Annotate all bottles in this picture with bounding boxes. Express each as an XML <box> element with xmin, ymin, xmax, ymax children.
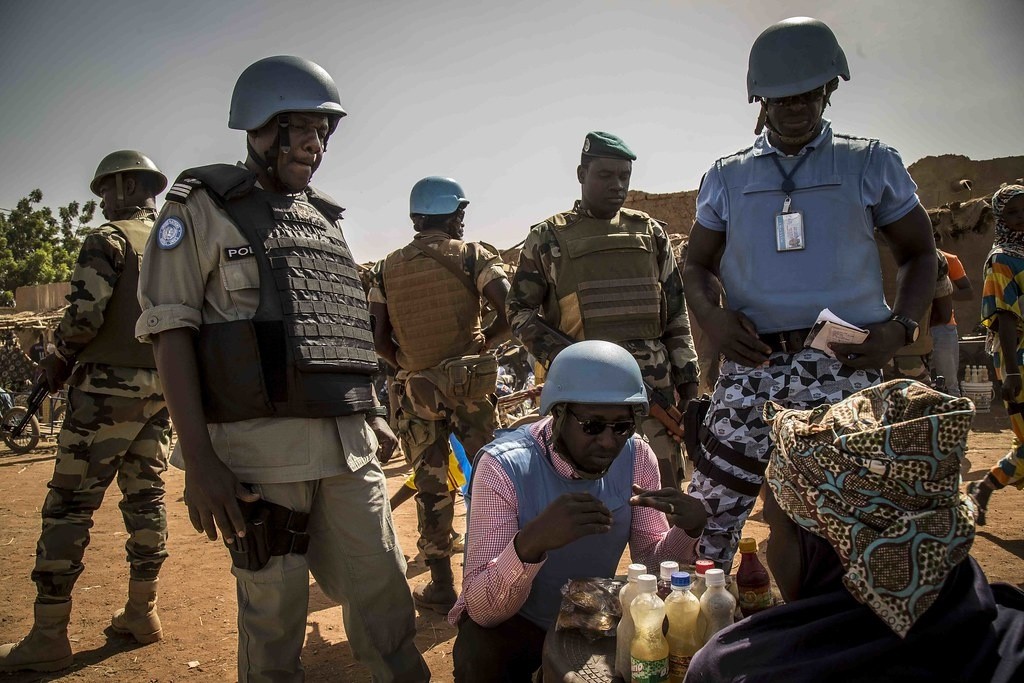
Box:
<box><xmin>700</xmin><ymin>569</ymin><xmax>736</xmax><ymax>643</ymax></box>
<box><xmin>655</xmin><ymin>561</ymin><xmax>679</xmax><ymax>637</ymax></box>
<box><xmin>664</xmin><ymin>571</ymin><xmax>704</xmax><ymax>683</ymax></box>
<box><xmin>614</xmin><ymin>564</ymin><xmax>646</xmax><ymax>683</ymax></box>
<box><xmin>965</xmin><ymin>365</ymin><xmax>988</xmax><ymax>383</ymax></box>
<box><xmin>736</xmin><ymin>538</ymin><xmax>773</xmax><ymax>618</ymax></box>
<box><xmin>630</xmin><ymin>574</ymin><xmax>670</xmax><ymax>683</ymax></box>
<box><xmin>689</xmin><ymin>560</ymin><xmax>714</xmax><ymax>637</ymax></box>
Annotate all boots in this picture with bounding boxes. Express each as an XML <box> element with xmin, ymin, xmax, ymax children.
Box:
<box><xmin>110</xmin><ymin>578</ymin><xmax>164</xmax><ymax>644</ymax></box>
<box><xmin>413</xmin><ymin>556</ymin><xmax>458</xmax><ymax>615</ymax></box>
<box><xmin>0</xmin><ymin>599</ymin><xmax>74</xmax><ymax>672</ymax></box>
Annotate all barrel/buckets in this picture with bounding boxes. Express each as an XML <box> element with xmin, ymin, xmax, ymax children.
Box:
<box><xmin>960</xmin><ymin>381</ymin><xmax>995</xmax><ymax>413</ymax></box>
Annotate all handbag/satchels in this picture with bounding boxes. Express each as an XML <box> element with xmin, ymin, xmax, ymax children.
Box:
<box><xmin>419</xmin><ymin>355</ymin><xmax>499</xmax><ymax>397</ymax></box>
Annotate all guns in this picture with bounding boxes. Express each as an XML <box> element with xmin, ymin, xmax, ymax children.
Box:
<box><xmin>517</xmin><ymin>305</ymin><xmax>685</xmax><ymax>438</ymax></box>
<box><xmin>4</xmin><ymin>371</ymin><xmax>50</xmax><ymax>439</ymax></box>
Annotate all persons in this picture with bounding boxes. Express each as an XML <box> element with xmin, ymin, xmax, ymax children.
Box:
<box><xmin>966</xmin><ymin>183</ymin><xmax>1024</xmax><ymax>526</ymax></box>
<box><xmin>505</xmin><ymin>131</ymin><xmax>701</xmax><ymax>526</ymax></box>
<box><xmin>788</xmin><ymin>235</ymin><xmax>802</xmax><ymax>246</ymax></box>
<box><xmin>29</xmin><ymin>333</ymin><xmax>50</xmax><ymax>362</ymax></box>
<box><xmin>679</xmin><ymin>20</ymin><xmax>937</xmax><ymax>617</ymax></box>
<box><xmin>136</xmin><ymin>53</ymin><xmax>436</xmax><ymax>683</ymax></box>
<box><xmin>445</xmin><ymin>337</ymin><xmax>708</xmax><ymax>683</ymax></box>
<box><xmin>0</xmin><ymin>149</ymin><xmax>175</xmax><ymax>675</ymax></box>
<box><xmin>924</xmin><ymin>225</ymin><xmax>974</xmax><ymax>395</ymax></box>
<box><xmin>366</xmin><ymin>178</ymin><xmax>513</xmax><ymax>618</ymax></box>
<box><xmin>681</xmin><ymin>372</ymin><xmax>1024</xmax><ymax>683</ymax></box>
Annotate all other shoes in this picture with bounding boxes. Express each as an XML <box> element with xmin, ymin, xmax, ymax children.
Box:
<box><xmin>965</xmin><ymin>481</ymin><xmax>991</xmax><ymax>525</ymax></box>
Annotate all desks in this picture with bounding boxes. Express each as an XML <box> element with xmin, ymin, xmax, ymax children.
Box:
<box><xmin>540</xmin><ymin>576</ymin><xmax>785</xmax><ymax>683</ymax></box>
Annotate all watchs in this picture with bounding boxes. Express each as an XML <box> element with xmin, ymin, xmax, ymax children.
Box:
<box><xmin>890</xmin><ymin>314</ymin><xmax>921</xmax><ymax>347</ymax></box>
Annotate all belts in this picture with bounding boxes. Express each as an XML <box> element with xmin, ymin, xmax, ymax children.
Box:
<box><xmin>755</xmin><ymin>328</ymin><xmax>810</xmax><ymax>354</ymax></box>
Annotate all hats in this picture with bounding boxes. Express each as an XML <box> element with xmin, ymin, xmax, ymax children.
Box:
<box><xmin>581</xmin><ymin>132</ymin><xmax>637</xmax><ymax>161</ymax></box>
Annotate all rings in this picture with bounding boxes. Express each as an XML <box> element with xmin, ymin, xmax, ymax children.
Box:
<box><xmin>668</xmin><ymin>504</ymin><xmax>675</xmax><ymax>513</ymax></box>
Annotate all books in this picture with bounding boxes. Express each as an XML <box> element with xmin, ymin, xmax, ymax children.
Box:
<box><xmin>803</xmin><ymin>307</ymin><xmax>870</xmax><ymax>360</ymax></box>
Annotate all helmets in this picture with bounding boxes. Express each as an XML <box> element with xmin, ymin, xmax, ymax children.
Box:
<box><xmin>539</xmin><ymin>339</ymin><xmax>651</xmax><ymax>417</ymax></box>
<box><xmin>746</xmin><ymin>17</ymin><xmax>850</xmax><ymax>102</ymax></box>
<box><xmin>90</xmin><ymin>149</ymin><xmax>168</xmax><ymax>196</ymax></box>
<box><xmin>228</xmin><ymin>56</ymin><xmax>347</xmax><ymax>130</ymax></box>
<box><xmin>409</xmin><ymin>177</ymin><xmax>469</xmax><ymax>214</ymax></box>
<box><xmin>748</xmin><ymin>17</ymin><xmax>850</xmax><ymax>103</ymax></box>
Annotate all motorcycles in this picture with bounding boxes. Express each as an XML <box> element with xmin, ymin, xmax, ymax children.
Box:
<box><xmin>0</xmin><ymin>386</ymin><xmax>40</xmax><ymax>453</ymax></box>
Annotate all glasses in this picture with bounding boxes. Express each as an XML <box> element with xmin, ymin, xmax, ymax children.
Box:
<box><xmin>565</xmin><ymin>408</ymin><xmax>635</xmax><ymax>437</ymax></box>
<box><xmin>766</xmin><ymin>83</ymin><xmax>827</xmax><ymax>107</ymax></box>
